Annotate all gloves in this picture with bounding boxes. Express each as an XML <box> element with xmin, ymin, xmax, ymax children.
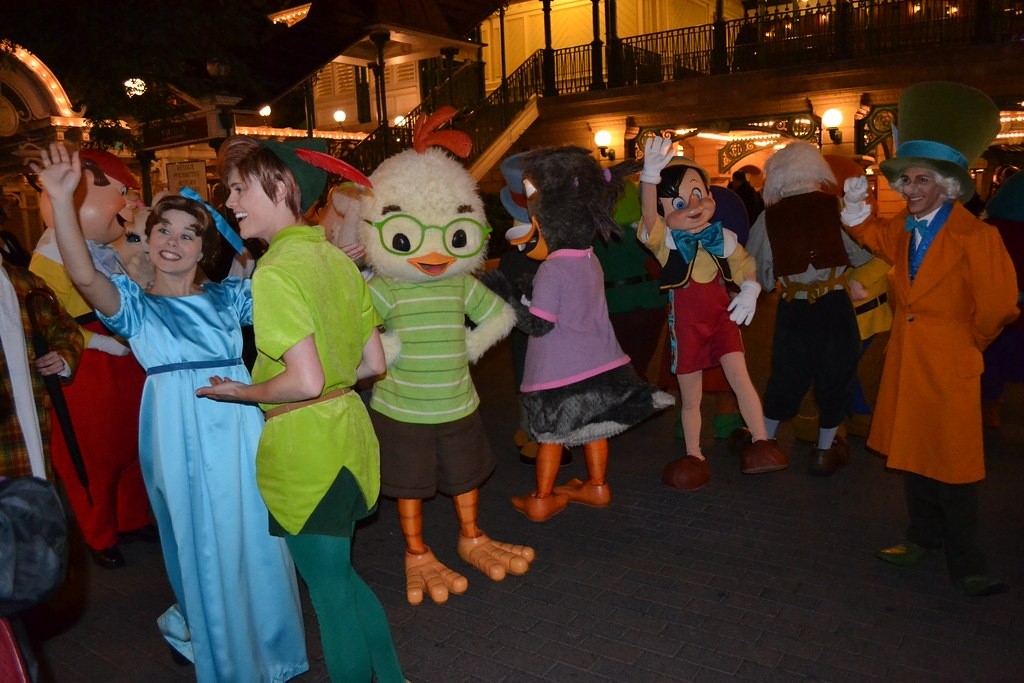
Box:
<box><xmin>843</xmin><ymin>176</ymin><xmax>869</xmax><ymax>203</ymax></box>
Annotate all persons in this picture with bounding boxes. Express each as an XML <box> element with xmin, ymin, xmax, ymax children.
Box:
<box><xmin>962</xmin><ymin>165</ymin><xmax>1017</xmax><ymax>221</ymax></box>
<box><xmin>725</xmin><ymin>168</ymin><xmax>756</xmax><ymax>221</ymax></box>
<box><xmin>0</xmin><ymin>207</ymin><xmax>84</xmax><ymax>493</ymax></box>
<box><xmin>36</xmin><ymin>140</ymin><xmax>365</xmax><ymax>683</ymax></box>
<box><xmin>735</xmin><ymin>22</ymin><xmax>762</xmax><ymax>63</ymax></box>
<box><xmin>195</xmin><ymin>138</ymin><xmax>414</xmax><ymax>683</ymax></box>
<box><xmin>0</xmin><ymin>476</ymin><xmax>67</xmax><ymax>683</ymax></box>
<box><xmin>840</xmin><ymin>80</ymin><xmax>1020</xmax><ymax>599</ymax></box>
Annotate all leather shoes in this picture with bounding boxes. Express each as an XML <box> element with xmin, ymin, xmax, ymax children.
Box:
<box><xmin>93</xmin><ymin>544</ymin><xmax>124</xmax><ymax>569</ymax></box>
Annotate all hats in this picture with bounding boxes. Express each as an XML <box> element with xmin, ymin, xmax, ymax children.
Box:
<box><xmin>879</xmin><ymin>81</ymin><xmax>1001</xmax><ymax>204</ymax></box>
<box><xmin>258</xmin><ymin>137</ymin><xmax>373</xmax><ymax>212</ymax></box>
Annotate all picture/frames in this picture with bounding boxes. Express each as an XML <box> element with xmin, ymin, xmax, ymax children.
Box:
<box><xmin>166</xmin><ymin>160</ymin><xmax>209</xmax><ymax>203</ymax></box>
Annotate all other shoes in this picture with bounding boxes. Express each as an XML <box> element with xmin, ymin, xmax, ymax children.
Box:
<box><xmin>877</xmin><ymin>542</ymin><xmax>930</xmax><ymax>568</ymax></box>
<box><xmin>956</xmin><ymin>574</ymin><xmax>1009</xmax><ymax>595</ymax></box>
<box><xmin>809</xmin><ymin>444</ymin><xmax>849</xmax><ymax>475</ymax></box>
<box><xmin>729</xmin><ymin>427</ymin><xmax>753</xmax><ymax>450</ymax></box>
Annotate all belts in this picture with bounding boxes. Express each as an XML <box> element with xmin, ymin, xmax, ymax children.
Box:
<box><xmin>265</xmin><ymin>386</ymin><xmax>353</xmax><ymax>420</ymax></box>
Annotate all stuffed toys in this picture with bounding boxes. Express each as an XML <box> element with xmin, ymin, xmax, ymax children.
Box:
<box><xmin>27</xmin><ymin>145</ymin><xmax>177</xmax><ymax>571</ymax></box>
<box><xmin>356</xmin><ymin>107</ymin><xmax>537</xmax><ymax>606</ymax></box>
<box><xmin>105</xmin><ymin>192</ymin><xmax>174</xmax><ymax>292</ymax></box>
<box><xmin>303</xmin><ymin>179</ymin><xmax>372</xmax><ymax>273</ymax></box>
<box><xmin>637</xmin><ymin>133</ymin><xmax>787</xmax><ymax>492</ymax></box>
<box><xmin>473</xmin><ymin>143</ymin><xmax>676</xmax><ymax>522</ymax></box>
<box><xmin>744</xmin><ymin>141</ymin><xmax>862</xmax><ymax>476</ymax></box>
<box><xmin>822</xmin><ymin>154</ymin><xmax>892</xmax><ymax>460</ymax></box>
<box><xmin>982</xmin><ymin>171</ymin><xmax>1024</xmax><ymax>428</ymax></box>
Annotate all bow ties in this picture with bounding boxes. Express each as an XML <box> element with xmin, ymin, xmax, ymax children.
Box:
<box><xmin>904</xmin><ymin>214</ymin><xmax>928</xmax><ymax>237</ymax></box>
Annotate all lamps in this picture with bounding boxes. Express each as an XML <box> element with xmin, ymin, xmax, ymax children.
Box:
<box><xmin>334</xmin><ymin>110</ymin><xmax>346</xmax><ymax>133</ymax></box>
<box><xmin>595</xmin><ymin>129</ymin><xmax>617</xmax><ymax>162</ymax></box>
<box><xmin>394</xmin><ymin>116</ymin><xmax>408</xmax><ymax>141</ymax></box>
<box><xmin>259</xmin><ymin>106</ymin><xmax>271</xmax><ymax>125</ymax></box>
<box><xmin>823</xmin><ymin>108</ymin><xmax>843</xmax><ymax>144</ymax></box>
